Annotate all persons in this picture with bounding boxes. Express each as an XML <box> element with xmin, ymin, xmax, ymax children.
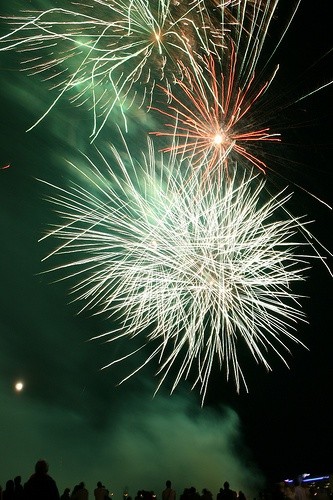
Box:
<box><xmin>180</xmin><ymin>481</ymin><xmax>246</xmax><ymax>500</ymax></box>
<box><xmin>60</xmin><ymin>487</ymin><xmax>71</xmax><ymax>500</ymax></box>
<box><xmin>94</xmin><ymin>481</ymin><xmax>107</xmax><ymax>500</ymax></box>
<box><xmin>122</xmin><ymin>488</ymin><xmax>158</xmax><ymax>500</ymax></box>
<box><xmin>0</xmin><ymin>479</ymin><xmax>19</xmax><ymax>500</ymax></box>
<box><xmin>13</xmin><ymin>475</ymin><xmax>24</xmax><ymax>500</ymax></box>
<box><xmin>79</xmin><ymin>481</ymin><xmax>89</xmax><ymax>500</ymax></box>
<box><xmin>162</xmin><ymin>480</ymin><xmax>177</xmax><ymax>500</ymax></box>
<box><xmin>102</xmin><ymin>485</ymin><xmax>112</xmax><ymax>500</ymax></box>
<box><xmin>70</xmin><ymin>484</ymin><xmax>86</xmax><ymax>500</ymax></box>
<box><xmin>254</xmin><ymin>474</ymin><xmax>333</xmax><ymax>500</ymax></box>
<box><xmin>24</xmin><ymin>458</ymin><xmax>60</xmax><ymax>500</ymax></box>
